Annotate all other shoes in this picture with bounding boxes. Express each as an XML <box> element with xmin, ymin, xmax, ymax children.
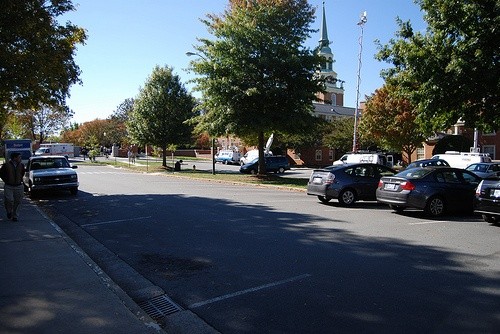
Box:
<box><xmin>7</xmin><ymin>216</ymin><xmax>18</xmax><ymax>222</ymax></box>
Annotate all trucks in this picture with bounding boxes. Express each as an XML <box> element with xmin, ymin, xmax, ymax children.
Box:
<box><xmin>73</xmin><ymin>146</ymin><xmax>82</xmax><ymax>157</ymax></box>
<box><xmin>333</xmin><ymin>153</ymin><xmax>394</xmax><ymax>169</ymax></box>
<box><xmin>239</xmin><ymin>149</ymin><xmax>273</xmax><ymax>166</ymax></box>
<box><xmin>35</xmin><ymin>142</ymin><xmax>75</xmax><ymax>161</ymax></box>
<box><xmin>4</xmin><ymin>138</ymin><xmax>33</xmax><ymax>166</ymax></box>
<box><xmin>214</xmin><ymin>150</ymin><xmax>241</xmax><ymax>165</ymax></box>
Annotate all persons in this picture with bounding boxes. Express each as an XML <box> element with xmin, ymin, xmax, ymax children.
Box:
<box><xmin>0</xmin><ymin>152</ymin><xmax>25</xmax><ymax>222</ymax></box>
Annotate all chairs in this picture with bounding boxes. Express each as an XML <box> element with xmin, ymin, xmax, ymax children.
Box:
<box><xmin>31</xmin><ymin>163</ymin><xmax>42</xmax><ymax>170</ymax></box>
<box><xmin>355</xmin><ymin>168</ymin><xmax>371</xmax><ymax>176</ymax></box>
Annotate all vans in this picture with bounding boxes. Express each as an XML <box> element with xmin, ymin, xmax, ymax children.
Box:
<box><xmin>239</xmin><ymin>154</ymin><xmax>290</xmax><ymax>174</ymax></box>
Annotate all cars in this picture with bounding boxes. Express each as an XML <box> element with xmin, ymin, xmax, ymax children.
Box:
<box><xmin>306</xmin><ymin>162</ymin><xmax>399</xmax><ymax>208</ymax></box>
<box><xmin>23</xmin><ymin>155</ymin><xmax>78</xmax><ymax>200</ymax></box>
<box><xmin>376</xmin><ymin>150</ymin><xmax>500</xmax><ymax>224</ymax></box>
